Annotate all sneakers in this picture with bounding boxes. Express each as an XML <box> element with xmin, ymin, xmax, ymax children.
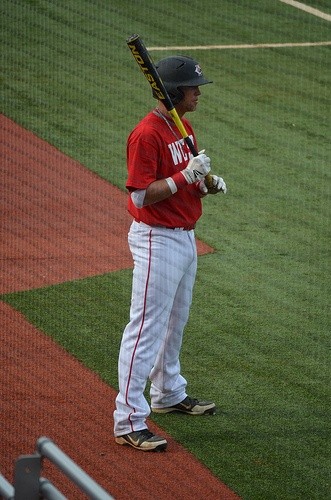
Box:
<box><xmin>115</xmin><ymin>429</ymin><xmax>168</xmax><ymax>452</ymax></box>
<box><xmin>152</xmin><ymin>396</ymin><xmax>216</xmax><ymax>416</ymax></box>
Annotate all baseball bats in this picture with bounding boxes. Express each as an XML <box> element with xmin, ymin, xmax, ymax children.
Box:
<box><xmin>127</xmin><ymin>33</ymin><xmax>220</xmax><ymax>193</ymax></box>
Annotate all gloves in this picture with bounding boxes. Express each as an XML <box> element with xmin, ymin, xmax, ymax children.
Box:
<box><xmin>199</xmin><ymin>175</ymin><xmax>227</xmax><ymax>194</ymax></box>
<box><xmin>180</xmin><ymin>149</ymin><xmax>211</xmax><ymax>185</ymax></box>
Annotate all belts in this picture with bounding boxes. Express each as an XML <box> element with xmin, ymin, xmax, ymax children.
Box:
<box><xmin>166</xmin><ymin>227</ymin><xmax>193</xmax><ymax>231</ymax></box>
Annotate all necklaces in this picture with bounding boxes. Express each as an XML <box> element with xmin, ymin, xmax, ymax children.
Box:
<box><xmin>155</xmin><ymin>107</ymin><xmax>189</xmax><ymax>154</ymax></box>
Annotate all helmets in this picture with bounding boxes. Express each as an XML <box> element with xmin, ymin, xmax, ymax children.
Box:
<box><xmin>154</xmin><ymin>55</ymin><xmax>213</xmax><ymax>102</ymax></box>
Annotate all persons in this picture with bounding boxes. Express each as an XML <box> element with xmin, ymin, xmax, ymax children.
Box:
<box><xmin>113</xmin><ymin>57</ymin><xmax>228</xmax><ymax>451</ymax></box>
